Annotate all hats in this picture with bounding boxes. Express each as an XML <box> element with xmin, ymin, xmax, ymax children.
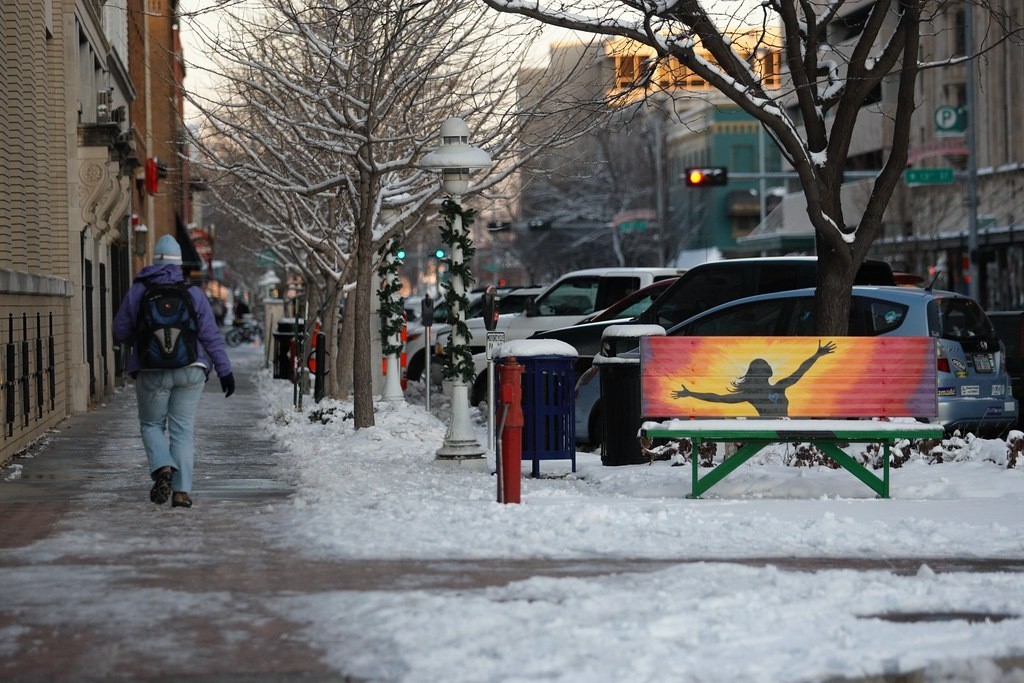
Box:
<box><xmin>153</xmin><ymin>233</ymin><xmax>182</xmax><ymax>265</ymax></box>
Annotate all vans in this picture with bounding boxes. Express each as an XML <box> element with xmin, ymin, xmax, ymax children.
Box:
<box><xmin>505</xmin><ymin>266</ymin><xmax>690</xmax><ymax>342</ymax></box>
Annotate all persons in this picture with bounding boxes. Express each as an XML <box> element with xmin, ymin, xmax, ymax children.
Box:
<box><xmin>233</xmin><ymin>295</ymin><xmax>250</xmax><ymax>319</ymax></box>
<box><xmin>208</xmin><ymin>296</ymin><xmax>228</xmax><ymax>327</ymax></box>
<box><xmin>112</xmin><ymin>234</ymin><xmax>235</xmax><ymax>508</ymax></box>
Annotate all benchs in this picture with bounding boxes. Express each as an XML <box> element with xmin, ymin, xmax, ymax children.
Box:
<box><xmin>639</xmin><ymin>334</ymin><xmax>945</xmax><ymax>498</ymax></box>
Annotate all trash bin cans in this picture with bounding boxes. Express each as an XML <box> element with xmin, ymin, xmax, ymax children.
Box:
<box><xmin>493</xmin><ymin>339</ymin><xmax>576</xmax><ymax>479</ymax></box>
<box><xmin>592</xmin><ymin>324</ymin><xmax>670</xmax><ymax>466</ymax></box>
<box><xmin>272</xmin><ymin>318</ymin><xmax>303</xmax><ymax>379</ymax></box>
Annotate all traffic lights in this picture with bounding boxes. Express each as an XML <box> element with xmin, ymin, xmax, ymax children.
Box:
<box><xmin>528</xmin><ymin>220</ymin><xmax>552</xmax><ymax>230</ymax></box>
<box><xmin>488</xmin><ymin>221</ymin><xmax>511</xmax><ymax>231</ymax></box>
<box><xmin>684</xmin><ymin>166</ymin><xmax>727</xmax><ymax>187</ymax></box>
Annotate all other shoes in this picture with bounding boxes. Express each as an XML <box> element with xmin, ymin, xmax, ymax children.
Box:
<box><xmin>149</xmin><ymin>466</ymin><xmax>172</xmax><ymax>505</ymax></box>
<box><xmin>171</xmin><ymin>491</ymin><xmax>193</xmax><ymax>507</ymax></box>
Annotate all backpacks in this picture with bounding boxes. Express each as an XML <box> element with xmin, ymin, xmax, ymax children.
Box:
<box><xmin>132</xmin><ymin>278</ymin><xmax>199</xmax><ymax>370</ymax></box>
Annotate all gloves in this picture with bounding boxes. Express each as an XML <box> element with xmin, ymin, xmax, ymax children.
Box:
<box><xmin>220</xmin><ymin>372</ymin><xmax>235</xmax><ymax>397</ymax></box>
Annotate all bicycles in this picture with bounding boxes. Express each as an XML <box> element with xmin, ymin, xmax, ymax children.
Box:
<box><xmin>225</xmin><ymin>320</ymin><xmax>263</xmax><ymax>348</ymax></box>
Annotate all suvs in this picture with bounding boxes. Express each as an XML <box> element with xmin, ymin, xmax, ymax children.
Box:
<box><xmin>525</xmin><ymin>256</ymin><xmax>898</xmax><ymax>388</ymax></box>
<box><xmin>405</xmin><ymin>289</ymin><xmax>512</xmax><ymax>381</ymax></box>
<box><xmin>432</xmin><ymin>288</ymin><xmax>546</xmax><ymax>368</ymax></box>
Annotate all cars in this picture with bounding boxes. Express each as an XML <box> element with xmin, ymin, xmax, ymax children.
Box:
<box><xmin>573</xmin><ymin>270</ymin><xmax>1021</xmax><ymax>449</ymax></box>
<box><xmin>574</xmin><ymin>277</ymin><xmax>682</xmax><ymax>325</ymax></box>
